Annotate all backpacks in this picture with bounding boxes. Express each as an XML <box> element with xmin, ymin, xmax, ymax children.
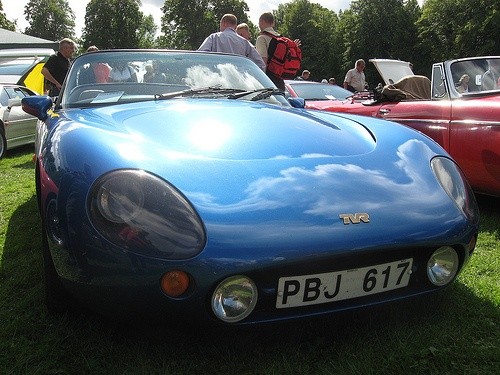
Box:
<box><xmin>261</xmin><ymin>31</ymin><xmax>303</xmax><ymax>80</ymax></box>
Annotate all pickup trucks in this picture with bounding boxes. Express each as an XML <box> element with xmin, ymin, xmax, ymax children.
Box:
<box><xmin>321</xmin><ymin>55</ymin><xmax>500</xmax><ymax>198</ymax></box>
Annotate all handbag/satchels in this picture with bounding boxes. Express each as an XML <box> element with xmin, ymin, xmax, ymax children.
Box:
<box><xmin>347</xmin><ymin>85</ymin><xmax>355</xmax><ymax>92</ymax></box>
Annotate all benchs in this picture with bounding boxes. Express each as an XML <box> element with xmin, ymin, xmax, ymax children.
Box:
<box><xmin>69</xmin><ymin>83</ymin><xmax>190</xmax><ymax>102</ymax></box>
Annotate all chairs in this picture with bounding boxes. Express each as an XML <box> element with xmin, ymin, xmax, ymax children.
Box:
<box><xmin>382</xmin><ymin>76</ymin><xmax>431</xmax><ymax>101</ymax></box>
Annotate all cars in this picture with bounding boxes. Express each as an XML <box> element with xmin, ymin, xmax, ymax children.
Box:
<box><xmin>0</xmin><ymin>85</ymin><xmax>41</xmax><ymax>158</ymax></box>
<box><xmin>284</xmin><ymin>80</ymin><xmax>356</xmax><ymax>111</ymax></box>
<box><xmin>22</xmin><ymin>49</ymin><xmax>479</xmax><ymax>332</ymax></box>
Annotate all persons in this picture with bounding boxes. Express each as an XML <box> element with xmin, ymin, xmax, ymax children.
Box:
<box><xmin>455</xmin><ymin>59</ymin><xmax>500</xmax><ymax>93</ymax></box>
<box><xmin>41</xmin><ymin>38</ymin><xmax>75</xmax><ymax>106</ymax></box>
<box><xmin>321</xmin><ymin>78</ymin><xmax>335</xmax><ymax>85</ymax></box>
<box><xmin>78</xmin><ymin>46</ymin><xmax>167</xmax><ymax>85</ymax></box>
<box><xmin>256</xmin><ymin>12</ymin><xmax>302</xmax><ymax>97</ymax></box>
<box><xmin>343</xmin><ymin>59</ymin><xmax>365</xmax><ymax>93</ymax></box>
<box><xmin>196</xmin><ymin>14</ymin><xmax>266</xmax><ymax>72</ymax></box>
<box><xmin>294</xmin><ymin>70</ymin><xmax>310</xmax><ymax>81</ymax></box>
